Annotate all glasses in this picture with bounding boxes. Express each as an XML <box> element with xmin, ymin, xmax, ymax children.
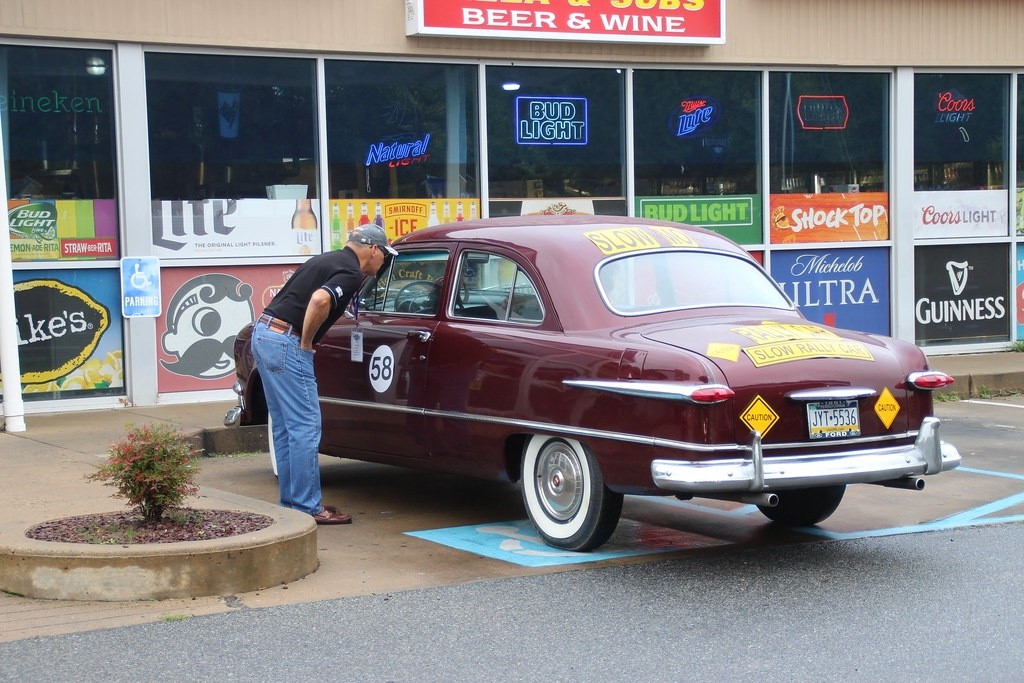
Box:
<box><xmin>370</xmin><ymin>243</ymin><xmax>388</xmax><ymax>256</ymax></box>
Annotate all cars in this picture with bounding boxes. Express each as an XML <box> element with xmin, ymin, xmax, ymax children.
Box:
<box><xmin>222</xmin><ymin>214</ymin><xmax>963</xmax><ymax>554</ymax></box>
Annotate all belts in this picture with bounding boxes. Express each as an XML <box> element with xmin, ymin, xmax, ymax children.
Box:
<box><xmin>259</xmin><ymin>315</ymin><xmax>302</xmax><ymax>336</ymax></box>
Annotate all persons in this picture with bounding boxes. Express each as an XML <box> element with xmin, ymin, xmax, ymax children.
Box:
<box><xmin>251</xmin><ymin>223</ymin><xmax>399</xmax><ymax>524</ymax></box>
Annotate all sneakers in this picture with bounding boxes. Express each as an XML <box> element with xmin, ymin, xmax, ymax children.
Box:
<box><xmin>314</xmin><ymin>506</ymin><xmax>352</xmax><ymax>524</ymax></box>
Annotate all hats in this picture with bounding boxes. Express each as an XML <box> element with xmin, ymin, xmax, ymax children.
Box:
<box><xmin>349</xmin><ymin>224</ymin><xmax>398</xmax><ymax>256</ymax></box>
